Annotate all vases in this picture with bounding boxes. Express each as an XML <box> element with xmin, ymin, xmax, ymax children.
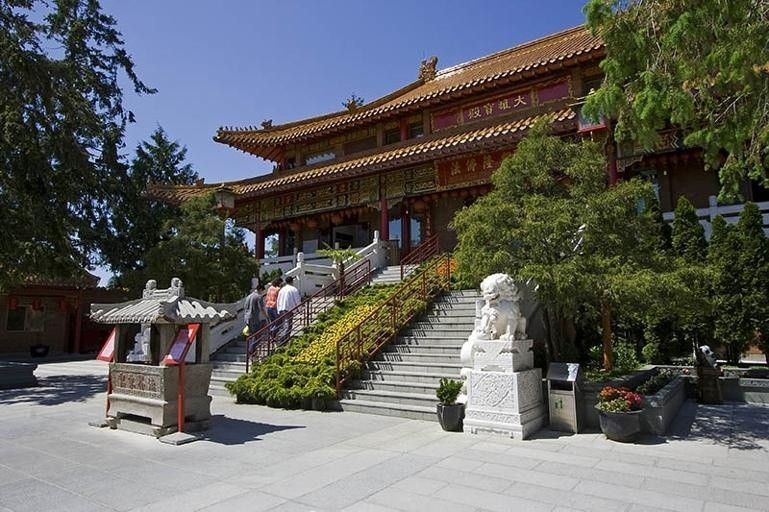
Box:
<box><xmin>595</xmin><ymin>402</ymin><xmax>647</xmax><ymax>444</ymax></box>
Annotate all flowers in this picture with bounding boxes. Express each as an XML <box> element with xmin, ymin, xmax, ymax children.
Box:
<box><xmin>599</xmin><ymin>385</ymin><xmax>643</xmax><ymax>412</ymax></box>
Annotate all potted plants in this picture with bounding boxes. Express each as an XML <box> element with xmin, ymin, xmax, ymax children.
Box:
<box><xmin>436</xmin><ymin>379</ymin><xmax>466</xmax><ymax>432</ymax></box>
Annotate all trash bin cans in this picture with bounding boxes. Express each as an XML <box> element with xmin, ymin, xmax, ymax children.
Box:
<box><xmin>546</xmin><ymin>362</ymin><xmax>588</xmax><ymax>433</ymax></box>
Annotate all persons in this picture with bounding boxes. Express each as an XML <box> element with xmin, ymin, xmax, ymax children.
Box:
<box><xmin>265</xmin><ymin>277</ymin><xmax>282</xmax><ymax>342</ymax></box>
<box><xmin>244</xmin><ymin>285</ymin><xmax>266</xmax><ymax>357</ymax></box>
<box><xmin>277</xmin><ymin>277</ymin><xmax>303</xmax><ymax>340</ymax></box>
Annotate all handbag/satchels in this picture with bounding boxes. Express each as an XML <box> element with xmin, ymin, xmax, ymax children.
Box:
<box><xmin>242</xmin><ymin>325</ymin><xmax>250</xmax><ymax>336</ymax></box>
